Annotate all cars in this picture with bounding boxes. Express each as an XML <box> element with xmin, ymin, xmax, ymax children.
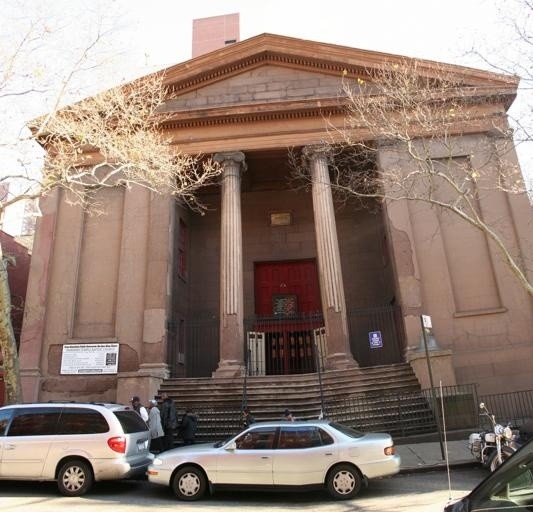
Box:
<box><xmin>442</xmin><ymin>441</ymin><xmax>533</xmax><ymax>511</ymax></box>
<box><xmin>147</xmin><ymin>407</ymin><xmax>402</xmax><ymax>500</ymax></box>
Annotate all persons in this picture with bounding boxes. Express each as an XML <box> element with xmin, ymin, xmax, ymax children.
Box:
<box><xmin>240</xmin><ymin>408</ymin><xmax>255</xmax><ymax>426</ymax></box>
<box><xmin>160</xmin><ymin>394</ymin><xmax>178</xmax><ymax>449</ymax></box>
<box><xmin>283</xmin><ymin>409</ymin><xmax>294</xmax><ymax>422</ymax></box>
<box><xmin>146</xmin><ymin>398</ymin><xmax>164</xmax><ymax>453</ymax></box>
<box><xmin>128</xmin><ymin>395</ymin><xmax>148</xmax><ymax>424</ymax></box>
<box><xmin>179</xmin><ymin>406</ymin><xmax>198</xmax><ymax>447</ymax></box>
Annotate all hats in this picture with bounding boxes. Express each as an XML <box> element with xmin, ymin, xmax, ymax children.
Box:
<box><xmin>284</xmin><ymin>409</ymin><xmax>293</xmax><ymax>418</ymax></box>
<box><xmin>128</xmin><ymin>396</ymin><xmax>139</xmax><ymax>403</ymax></box>
<box><xmin>150</xmin><ymin>400</ymin><xmax>157</xmax><ymax>407</ymax></box>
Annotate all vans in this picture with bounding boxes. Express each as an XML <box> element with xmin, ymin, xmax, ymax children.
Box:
<box><xmin>0</xmin><ymin>403</ymin><xmax>155</xmax><ymax>496</ymax></box>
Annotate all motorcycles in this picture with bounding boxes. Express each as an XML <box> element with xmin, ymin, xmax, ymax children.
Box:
<box><xmin>464</xmin><ymin>403</ymin><xmax>516</xmax><ymax>473</ymax></box>
